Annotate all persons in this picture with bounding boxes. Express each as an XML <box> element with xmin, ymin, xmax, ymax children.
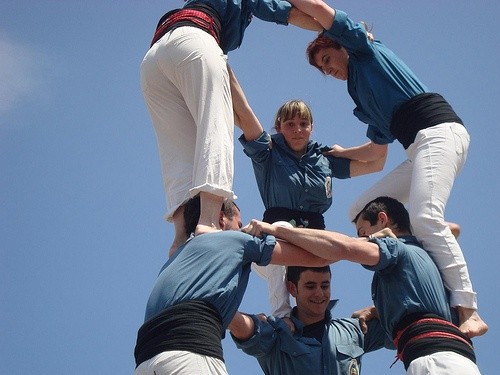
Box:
<box><xmin>139</xmin><ymin>1</ymin><xmax>376</xmax><ymax>258</ymax></box>
<box><xmin>134</xmin><ymin>193</ymin><xmax>399</xmax><ymax>375</ymax></box>
<box><xmin>286</xmin><ymin>0</ymin><xmax>490</xmax><ymax>340</ymax></box>
<box><xmin>226</xmin><ymin>60</ymin><xmax>389</xmax><ymax>333</ymax></box>
<box><xmin>240</xmin><ymin>197</ymin><xmax>482</xmax><ymax>375</ymax></box>
<box><xmin>229</xmin><ymin>266</ymin><xmax>392</xmax><ymax>375</ymax></box>
